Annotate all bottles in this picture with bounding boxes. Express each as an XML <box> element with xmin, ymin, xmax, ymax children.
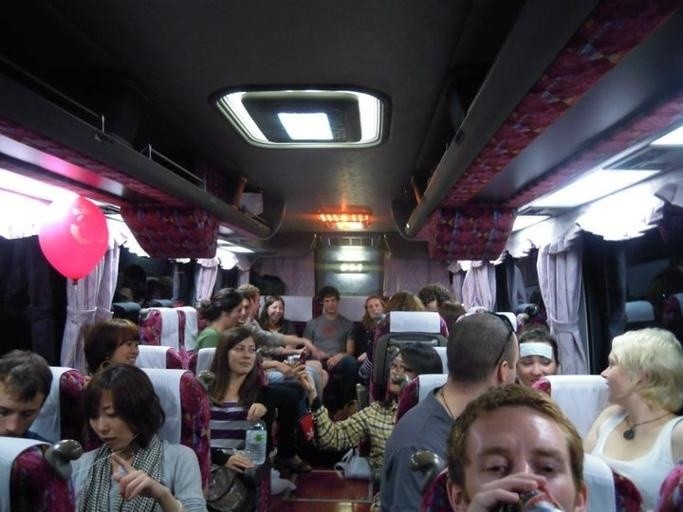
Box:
<box><xmin>245</xmin><ymin>419</ymin><xmax>268</xmax><ymax>465</ymax></box>
<box><xmin>511</xmin><ymin>485</ymin><xmax>566</xmax><ymax>512</ymax></box>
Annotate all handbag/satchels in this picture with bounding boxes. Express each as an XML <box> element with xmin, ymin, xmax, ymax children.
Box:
<box><xmin>207</xmin><ymin>466</ymin><xmax>250</xmax><ymax>512</ymax></box>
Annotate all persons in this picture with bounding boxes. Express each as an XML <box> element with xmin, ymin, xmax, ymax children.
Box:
<box><xmin>190</xmin><ymin>271</ymin><xmax>329</xmax><ymax>511</ymax></box>
<box><xmin>3</xmin><ymin>317</ymin><xmax>210</xmax><ymax>512</ymax></box>
<box><xmin>294</xmin><ymin>282</ymin><xmax>683</xmax><ymax>511</ymax></box>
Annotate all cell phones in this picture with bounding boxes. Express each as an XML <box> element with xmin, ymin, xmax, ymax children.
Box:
<box><xmin>301</xmin><ymin>350</ymin><xmax>306</xmax><ymax>366</ymax></box>
